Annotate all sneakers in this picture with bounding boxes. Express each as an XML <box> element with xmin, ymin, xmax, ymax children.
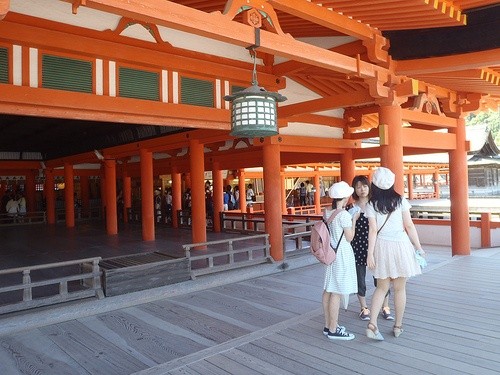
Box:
<box><xmin>381</xmin><ymin>306</ymin><xmax>394</xmax><ymax>321</ymax></box>
<box><xmin>322</xmin><ymin>326</ymin><xmax>355</xmax><ymax>341</ymax></box>
<box><xmin>359</xmin><ymin>308</ymin><xmax>370</xmax><ymax>320</ymax></box>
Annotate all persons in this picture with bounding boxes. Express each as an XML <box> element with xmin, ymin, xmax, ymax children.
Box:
<box><xmin>116</xmin><ymin>189</ymin><xmax>137</xmax><ymax>221</ymax></box>
<box><xmin>223</xmin><ymin>184</ymin><xmax>240</xmax><ymax>211</ymax></box>
<box><xmin>182</xmin><ymin>188</ymin><xmax>192</xmax><ymax>213</ymax></box>
<box><xmin>205</xmin><ymin>180</ymin><xmax>213</xmax><ymax>216</ymax></box>
<box><xmin>154</xmin><ymin>187</ymin><xmax>172</xmax><ymax>213</ymax></box>
<box><xmin>4</xmin><ymin>189</ymin><xmax>27</xmax><ymax>217</ymax></box>
<box><xmin>297</xmin><ymin>166</ymin><xmax>428</xmax><ymax>341</ymax></box>
<box><xmin>246</xmin><ymin>183</ymin><xmax>256</xmax><ymax>205</ymax></box>
<box><xmin>74</xmin><ymin>192</ymin><xmax>81</xmax><ymax>208</ymax></box>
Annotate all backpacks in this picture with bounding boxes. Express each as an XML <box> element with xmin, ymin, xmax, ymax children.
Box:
<box><xmin>310</xmin><ymin>208</ymin><xmax>345</xmax><ymax>266</ymax></box>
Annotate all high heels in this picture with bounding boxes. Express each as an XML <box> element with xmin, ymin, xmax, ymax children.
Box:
<box><xmin>393</xmin><ymin>325</ymin><xmax>404</xmax><ymax>338</ymax></box>
<box><xmin>366</xmin><ymin>323</ymin><xmax>385</xmax><ymax>341</ymax></box>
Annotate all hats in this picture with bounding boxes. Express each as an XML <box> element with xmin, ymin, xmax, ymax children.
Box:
<box><xmin>371</xmin><ymin>167</ymin><xmax>396</xmax><ymax>190</ymax></box>
<box><xmin>328</xmin><ymin>181</ymin><xmax>354</xmax><ymax>199</ymax></box>
